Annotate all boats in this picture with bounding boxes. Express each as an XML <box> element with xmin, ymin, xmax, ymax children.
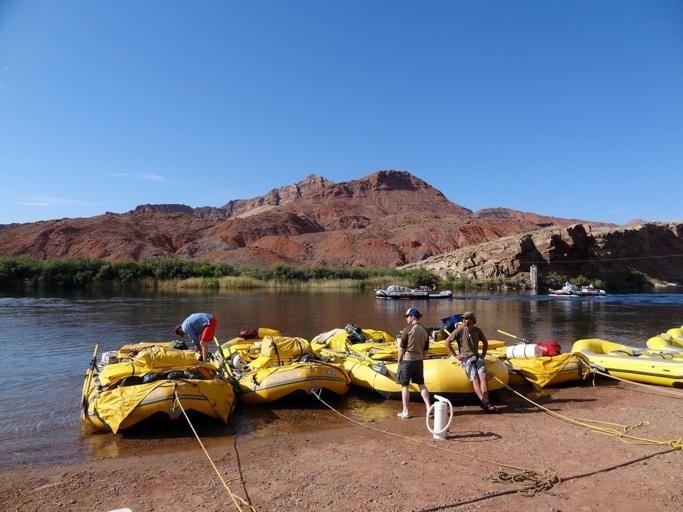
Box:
<box><xmin>548</xmin><ymin>282</ymin><xmax>580</xmax><ymax>295</ymax></box>
<box><xmin>311</xmin><ymin>328</ymin><xmax>509</xmax><ymax>397</ymax></box>
<box><xmin>374</xmin><ymin>285</ymin><xmax>428</xmax><ymax>300</ymax></box>
<box><xmin>647</xmin><ymin>325</ymin><xmax>683</xmax><ymax>353</ymax></box>
<box><xmin>547</xmin><ymin>294</ymin><xmax>579</xmax><ymax>301</ymax></box>
<box><xmin>82</xmin><ymin>342</ymin><xmax>233</xmax><ymax>431</ymax></box>
<box><xmin>577</xmin><ymin>284</ymin><xmax>606</xmax><ymax>296</ymax></box>
<box><xmin>570</xmin><ymin>336</ymin><xmax>683</xmax><ymax>389</ymax></box>
<box><xmin>418</xmin><ymin>286</ymin><xmax>451</xmax><ymax>298</ymax></box>
<box><xmin>216</xmin><ymin>329</ymin><xmax>348</xmax><ymax>404</ymax></box>
<box><xmin>443</xmin><ymin>335</ymin><xmax>586</xmax><ymax>388</ymax></box>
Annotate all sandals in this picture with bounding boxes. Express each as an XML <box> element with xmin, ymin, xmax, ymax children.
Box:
<box><xmin>480</xmin><ymin>401</ymin><xmax>498</xmax><ymax>412</ymax></box>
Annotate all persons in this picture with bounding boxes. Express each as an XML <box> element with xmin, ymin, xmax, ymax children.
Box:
<box><xmin>394</xmin><ymin>308</ymin><xmax>433</xmax><ymax>420</ymax></box>
<box><xmin>173</xmin><ymin>311</ymin><xmax>218</xmax><ymax>362</ymax></box>
<box><xmin>445</xmin><ymin>310</ymin><xmax>500</xmax><ymax>414</ymax></box>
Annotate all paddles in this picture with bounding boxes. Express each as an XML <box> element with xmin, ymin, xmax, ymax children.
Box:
<box><xmin>213</xmin><ymin>335</ymin><xmax>240</xmax><ymax>392</ymax></box>
<box><xmin>82</xmin><ymin>335</ymin><xmax>100</xmax><ymax>420</ymax></box>
<box><xmin>321</xmin><ymin>345</ymin><xmax>388</xmax><ymax>375</ymax></box>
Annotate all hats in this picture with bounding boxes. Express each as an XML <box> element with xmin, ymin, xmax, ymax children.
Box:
<box><xmin>403</xmin><ymin>307</ymin><xmax>419</xmax><ymax>318</ymax></box>
<box><xmin>174</xmin><ymin>323</ymin><xmax>181</xmax><ymax>335</ymax></box>
<box><xmin>460</xmin><ymin>311</ymin><xmax>473</xmax><ymax>319</ymax></box>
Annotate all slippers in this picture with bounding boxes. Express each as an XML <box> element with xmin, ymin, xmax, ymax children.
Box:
<box><xmin>396</xmin><ymin>411</ymin><xmax>409</xmax><ymax>417</ymax></box>
<box><xmin>429</xmin><ymin>414</ymin><xmax>433</xmax><ymax>417</ymax></box>
